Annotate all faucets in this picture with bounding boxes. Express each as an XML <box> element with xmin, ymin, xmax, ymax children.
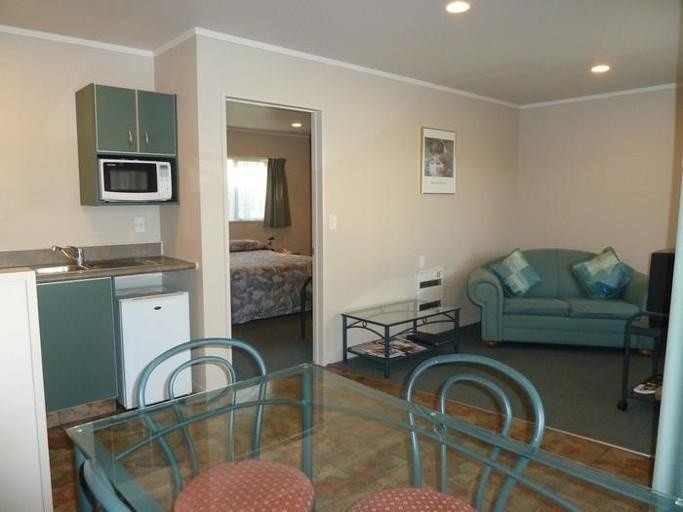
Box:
<box><xmin>50</xmin><ymin>244</ymin><xmax>89</xmax><ymax>269</ymax></box>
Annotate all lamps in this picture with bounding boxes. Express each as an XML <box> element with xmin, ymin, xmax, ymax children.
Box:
<box><xmin>268</xmin><ymin>237</ymin><xmax>276</xmax><ymax>251</ymax></box>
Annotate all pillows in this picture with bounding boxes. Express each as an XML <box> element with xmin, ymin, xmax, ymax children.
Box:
<box><xmin>570</xmin><ymin>246</ymin><xmax>634</xmax><ymax>300</ymax></box>
<box><xmin>488</xmin><ymin>248</ymin><xmax>543</xmax><ymax>298</ymax></box>
<box><xmin>229</xmin><ymin>238</ymin><xmax>271</xmax><ymax>251</ymax></box>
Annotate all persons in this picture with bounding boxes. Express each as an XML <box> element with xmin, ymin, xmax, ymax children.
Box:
<box><xmin>427</xmin><ymin>138</ymin><xmax>449</xmax><ymax>177</ymax></box>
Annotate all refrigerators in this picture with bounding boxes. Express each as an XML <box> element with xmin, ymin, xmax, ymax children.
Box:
<box><xmin>111</xmin><ymin>283</ymin><xmax>193</xmax><ymax>411</ymax></box>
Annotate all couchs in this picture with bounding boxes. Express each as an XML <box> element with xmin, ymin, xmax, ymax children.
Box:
<box><xmin>466</xmin><ymin>248</ymin><xmax>655</xmax><ymax>356</ymax></box>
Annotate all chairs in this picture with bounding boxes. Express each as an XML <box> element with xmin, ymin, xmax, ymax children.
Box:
<box><xmin>346</xmin><ymin>353</ymin><xmax>545</xmax><ymax>512</ymax></box>
<box><xmin>136</xmin><ymin>337</ymin><xmax>315</xmax><ymax>512</ymax></box>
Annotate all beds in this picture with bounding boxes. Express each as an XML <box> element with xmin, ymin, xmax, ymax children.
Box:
<box><xmin>229</xmin><ymin>250</ymin><xmax>313</xmax><ymax>325</ymax></box>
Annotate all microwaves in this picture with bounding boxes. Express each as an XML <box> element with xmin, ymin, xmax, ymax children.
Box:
<box><xmin>97</xmin><ymin>158</ymin><xmax>173</xmax><ymax>202</ymax></box>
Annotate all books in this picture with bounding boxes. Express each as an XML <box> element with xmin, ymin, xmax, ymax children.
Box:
<box><xmin>360</xmin><ymin>335</ymin><xmax>428</xmax><ymax>360</ymax></box>
<box><xmin>633</xmin><ymin>374</ymin><xmax>662</xmax><ymax>396</ymax></box>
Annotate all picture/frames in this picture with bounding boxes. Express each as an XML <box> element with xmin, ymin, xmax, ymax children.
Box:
<box><xmin>420</xmin><ymin>127</ymin><xmax>458</xmax><ymax>194</ymax></box>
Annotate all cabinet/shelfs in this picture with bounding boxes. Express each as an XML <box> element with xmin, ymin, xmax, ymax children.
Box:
<box><xmin>36</xmin><ymin>276</ymin><xmax>121</xmax><ymax>430</ymax></box>
<box><xmin>75</xmin><ymin>82</ymin><xmax>181</xmax><ymax>206</ymax></box>
<box><xmin>0</xmin><ymin>266</ymin><xmax>54</xmax><ymax>512</ymax></box>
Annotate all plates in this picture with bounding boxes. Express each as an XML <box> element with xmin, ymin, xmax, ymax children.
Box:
<box><xmin>632</xmin><ymin>383</ymin><xmax>657</xmax><ymax>396</ymax></box>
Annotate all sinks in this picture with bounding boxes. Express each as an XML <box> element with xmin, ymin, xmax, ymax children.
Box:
<box><xmin>33</xmin><ymin>255</ymin><xmax>154</xmax><ymax>276</ymax></box>
